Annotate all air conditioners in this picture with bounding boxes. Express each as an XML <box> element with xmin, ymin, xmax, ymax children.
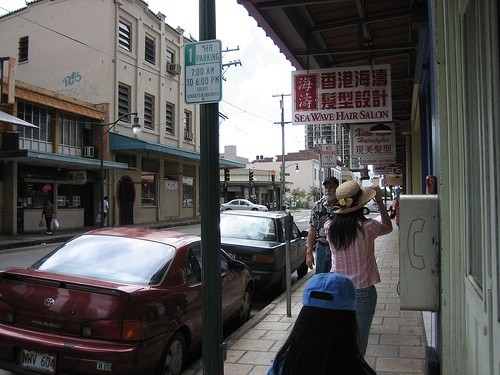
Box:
<box><xmin>168</xmin><ymin>63</ymin><xmax>181</xmax><ymax>74</ymax></box>
<box><xmin>84</xmin><ymin>147</ymin><xmax>94</xmax><ymax>157</ymax></box>
<box><xmin>184</xmin><ymin>132</ymin><xmax>193</xmax><ymax>139</ymax></box>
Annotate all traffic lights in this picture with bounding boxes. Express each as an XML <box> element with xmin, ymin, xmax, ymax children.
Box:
<box><xmin>272</xmin><ymin>172</ymin><xmax>275</xmax><ymax>181</ymax></box>
<box><xmin>224</xmin><ymin>168</ymin><xmax>230</xmax><ymax>181</ymax></box>
<box><xmin>249</xmin><ymin>171</ymin><xmax>254</xmax><ymax>181</ymax></box>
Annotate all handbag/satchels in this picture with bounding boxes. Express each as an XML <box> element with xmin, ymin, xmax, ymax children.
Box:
<box><xmin>53</xmin><ymin>218</ymin><xmax>59</xmax><ymax>231</ymax></box>
<box><xmin>39</xmin><ymin>218</ymin><xmax>45</xmax><ymax>226</ymax></box>
<box><xmin>96</xmin><ymin>213</ymin><xmax>102</xmax><ymax>222</ymax></box>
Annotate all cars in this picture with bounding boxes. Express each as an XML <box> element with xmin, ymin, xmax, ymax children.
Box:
<box><xmin>363</xmin><ymin>196</ymin><xmax>393</xmax><ymax>215</ymax></box>
<box><xmin>0</xmin><ymin>226</ymin><xmax>255</xmax><ymax>375</ymax></box>
<box><xmin>220</xmin><ymin>199</ymin><xmax>268</xmax><ymax>211</ymax></box>
<box><xmin>219</xmin><ymin>209</ymin><xmax>309</xmax><ymax>299</ymax></box>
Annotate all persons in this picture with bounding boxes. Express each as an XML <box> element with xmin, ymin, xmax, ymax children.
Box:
<box><xmin>103</xmin><ymin>196</ymin><xmax>109</xmax><ymax>226</ymax></box>
<box><xmin>323</xmin><ymin>180</ymin><xmax>393</xmax><ymax>358</ymax></box>
<box><xmin>268</xmin><ymin>271</ymin><xmax>377</xmax><ymax>375</ymax></box>
<box><xmin>306</xmin><ymin>176</ymin><xmax>339</xmax><ymax>275</ymax></box>
<box><xmin>41</xmin><ymin>199</ymin><xmax>56</xmax><ymax>234</ymax></box>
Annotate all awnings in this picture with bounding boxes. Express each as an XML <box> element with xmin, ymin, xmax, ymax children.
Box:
<box><xmin>0</xmin><ymin>110</ymin><xmax>39</xmax><ymax>128</ymax></box>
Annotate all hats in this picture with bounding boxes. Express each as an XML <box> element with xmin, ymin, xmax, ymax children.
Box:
<box><xmin>323</xmin><ymin>176</ymin><xmax>339</xmax><ymax>185</ymax></box>
<box><xmin>302</xmin><ymin>272</ymin><xmax>357</xmax><ymax>310</ymax></box>
<box><xmin>331</xmin><ymin>180</ymin><xmax>377</xmax><ymax>214</ymax></box>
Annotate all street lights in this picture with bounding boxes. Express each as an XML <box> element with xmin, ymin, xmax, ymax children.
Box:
<box><xmin>280</xmin><ymin>163</ymin><xmax>299</xmax><ymax>210</ymax></box>
<box><xmin>100</xmin><ymin>112</ymin><xmax>141</xmax><ymax>229</ymax></box>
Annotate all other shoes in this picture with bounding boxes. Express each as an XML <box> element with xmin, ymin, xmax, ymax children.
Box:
<box><xmin>46</xmin><ymin>231</ymin><xmax>52</xmax><ymax>234</ymax></box>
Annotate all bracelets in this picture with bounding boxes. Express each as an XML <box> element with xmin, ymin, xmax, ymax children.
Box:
<box><xmin>378</xmin><ymin>200</ymin><xmax>383</xmax><ymax>203</ymax></box>
